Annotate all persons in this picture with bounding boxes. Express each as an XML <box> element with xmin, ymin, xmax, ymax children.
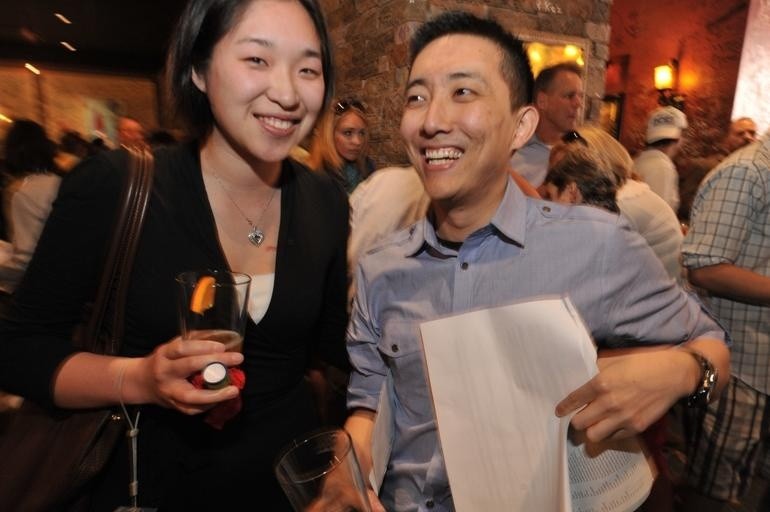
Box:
<box><xmin>510</xmin><ymin>64</ymin><xmax>588</xmax><ymax>189</ymax></box>
<box><xmin>308</xmin><ymin>13</ymin><xmax>730</xmax><ymax>512</ymax></box>
<box><xmin>0</xmin><ymin>118</ymin><xmax>63</xmax><ymax>294</ymax></box>
<box><xmin>716</xmin><ymin>117</ymin><xmax>756</xmax><ymax>157</ymax></box>
<box><xmin>543</xmin><ymin>125</ymin><xmax>683</xmax><ymax>285</ymax></box>
<box><xmin>541</xmin><ymin>153</ymin><xmax>616</xmax><ymax>215</ymax></box>
<box><xmin>0</xmin><ymin>0</ymin><xmax>349</xmax><ymax>512</ymax></box>
<box><xmin>633</xmin><ymin>108</ymin><xmax>685</xmax><ymax>221</ymax></box>
<box><xmin>685</xmin><ymin>128</ymin><xmax>770</xmax><ymax>512</ymax></box>
<box><xmin>316</xmin><ymin>97</ymin><xmax>387</xmax><ymax>191</ymax></box>
<box><xmin>113</xmin><ymin>114</ymin><xmax>147</xmax><ymax>151</ymax></box>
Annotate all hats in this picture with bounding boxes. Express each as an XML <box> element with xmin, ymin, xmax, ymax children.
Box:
<box><xmin>646</xmin><ymin>107</ymin><xmax>688</xmax><ymax>144</ymax></box>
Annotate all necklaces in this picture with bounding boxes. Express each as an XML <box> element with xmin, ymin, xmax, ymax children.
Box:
<box><xmin>206</xmin><ymin>144</ymin><xmax>282</xmax><ymax>247</ymax></box>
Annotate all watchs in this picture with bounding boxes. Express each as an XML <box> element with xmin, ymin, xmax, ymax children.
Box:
<box><xmin>679</xmin><ymin>342</ymin><xmax>716</xmax><ymax>407</ymax></box>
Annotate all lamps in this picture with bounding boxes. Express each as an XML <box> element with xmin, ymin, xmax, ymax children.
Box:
<box><xmin>653</xmin><ymin>63</ymin><xmax>673</xmax><ymax>104</ymax></box>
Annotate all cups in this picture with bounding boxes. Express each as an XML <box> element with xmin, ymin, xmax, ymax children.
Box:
<box><xmin>276</xmin><ymin>428</ymin><xmax>372</xmax><ymax>512</ymax></box>
<box><xmin>175</xmin><ymin>272</ymin><xmax>251</xmax><ymax>369</ymax></box>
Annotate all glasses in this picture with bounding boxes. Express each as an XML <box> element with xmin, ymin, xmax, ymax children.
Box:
<box><xmin>562</xmin><ymin>130</ymin><xmax>590</xmax><ymax>147</ymax></box>
<box><xmin>336</xmin><ymin>99</ymin><xmax>365</xmax><ymax>114</ymax></box>
